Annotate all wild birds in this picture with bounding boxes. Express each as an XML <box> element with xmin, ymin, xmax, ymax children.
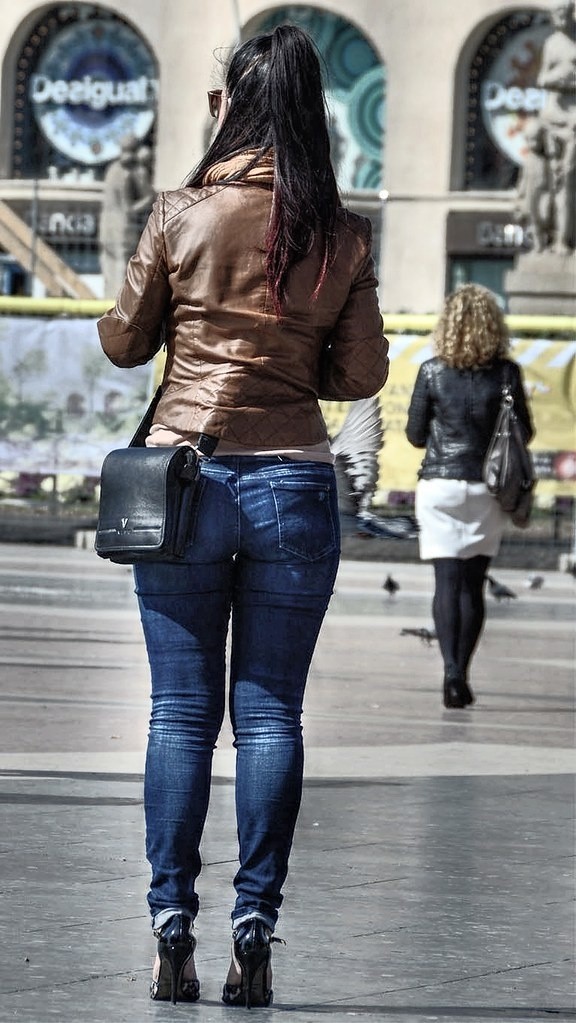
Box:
<box><xmin>381</xmin><ymin>573</ymin><xmax>544</xmax><ymax>607</ymax></box>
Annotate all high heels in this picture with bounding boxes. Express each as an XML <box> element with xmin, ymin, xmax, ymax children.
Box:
<box><xmin>222</xmin><ymin>917</ymin><xmax>274</xmax><ymax>1010</ymax></box>
<box><xmin>148</xmin><ymin>914</ymin><xmax>201</xmax><ymax>1006</ymax></box>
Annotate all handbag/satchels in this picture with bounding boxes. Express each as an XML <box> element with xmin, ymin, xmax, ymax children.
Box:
<box><xmin>94</xmin><ymin>445</ymin><xmax>201</xmax><ymax>562</ymax></box>
<box><xmin>483</xmin><ymin>359</ymin><xmax>534</xmax><ymax>531</ymax></box>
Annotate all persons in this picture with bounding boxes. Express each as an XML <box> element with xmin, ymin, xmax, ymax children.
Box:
<box><xmin>95</xmin><ymin>23</ymin><xmax>391</xmax><ymax>1013</ymax></box>
<box><xmin>403</xmin><ymin>285</ymin><xmax>536</xmax><ymax>711</ymax></box>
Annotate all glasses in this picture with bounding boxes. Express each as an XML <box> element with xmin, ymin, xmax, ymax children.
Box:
<box><xmin>207</xmin><ymin>89</ymin><xmax>230</xmax><ymax>118</ymax></box>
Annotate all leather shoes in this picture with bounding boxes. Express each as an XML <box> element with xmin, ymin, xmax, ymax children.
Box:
<box><xmin>444</xmin><ymin>678</ymin><xmax>473</xmax><ymax>708</ymax></box>
